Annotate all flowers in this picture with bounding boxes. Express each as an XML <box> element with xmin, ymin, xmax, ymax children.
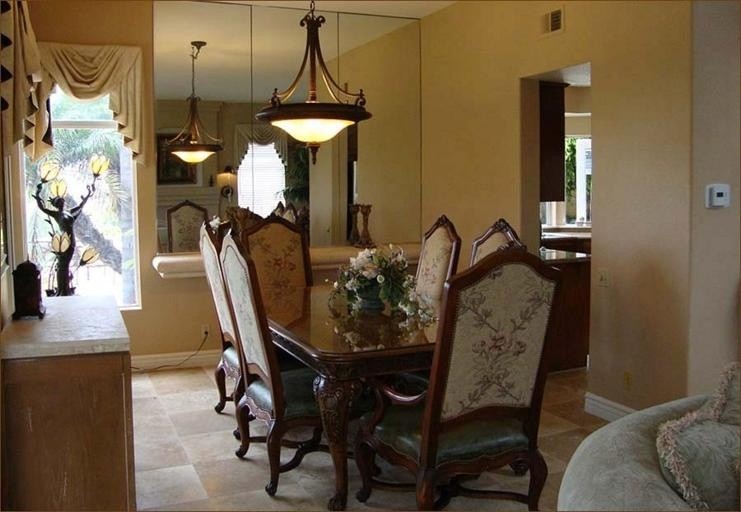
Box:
<box><xmin>31</xmin><ymin>158</ymin><xmax>110</xmax><ymax>296</ymax></box>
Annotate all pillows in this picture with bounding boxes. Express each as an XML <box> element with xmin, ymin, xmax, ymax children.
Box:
<box><xmin>656</xmin><ymin>362</ymin><xmax>741</xmax><ymax>512</ymax></box>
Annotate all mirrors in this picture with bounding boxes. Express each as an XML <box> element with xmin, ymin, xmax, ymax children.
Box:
<box><xmin>153</xmin><ymin>0</ymin><xmax>422</xmax><ymax>253</ymax></box>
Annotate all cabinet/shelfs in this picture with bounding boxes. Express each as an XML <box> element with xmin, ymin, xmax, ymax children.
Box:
<box><xmin>540</xmin><ymin>241</ymin><xmax>591</xmax><ymax>375</ymax></box>
<box><xmin>540</xmin><ymin>81</ymin><xmax>570</xmax><ymax>202</ymax></box>
<box><xmin>0</xmin><ymin>352</ymin><xmax>137</xmax><ymax>512</ymax></box>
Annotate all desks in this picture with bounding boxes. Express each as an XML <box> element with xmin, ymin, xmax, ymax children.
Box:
<box><xmin>258</xmin><ymin>282</ymin><xmax>438</xmax><ymax>510</ymax></box>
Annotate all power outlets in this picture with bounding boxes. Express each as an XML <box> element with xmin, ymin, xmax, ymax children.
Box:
<box><xmin>200</xmin><ymin>324</ymin><xmax>210</xmax><ymax>339</ymax></box>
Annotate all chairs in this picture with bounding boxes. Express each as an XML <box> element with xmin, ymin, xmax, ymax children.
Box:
<box><xmin>355</xmin><ymin>240</ymin><xmax>561</xmax><ymax>510</ymax></box>
<box><xmin>215</xmin><ymin>227</ymin><xmax>377</xmax><ymax>498</ymax></box>
<box><xmin>557</xmin><ymin>393</ymin><xmax>713</xmax><ymax>512</ymax></box>
<box><xmin>471</xmin><ymin>216</ymin><xmax>527</xmax><ymax>268</ymax></box>
<box><xmin>197</xmin><ymin>217</ymin><xmax>302</xmax><ymax>443</ymax></box>
<box><xmin>242</xmin><ymin>212</ymin><xmax>308</xmax><ymax>254</ymax></box>
<box><xmin>415</xmin><ymin>215</ymin><xmax>461</xmax><ymax>296</ymax></box>
